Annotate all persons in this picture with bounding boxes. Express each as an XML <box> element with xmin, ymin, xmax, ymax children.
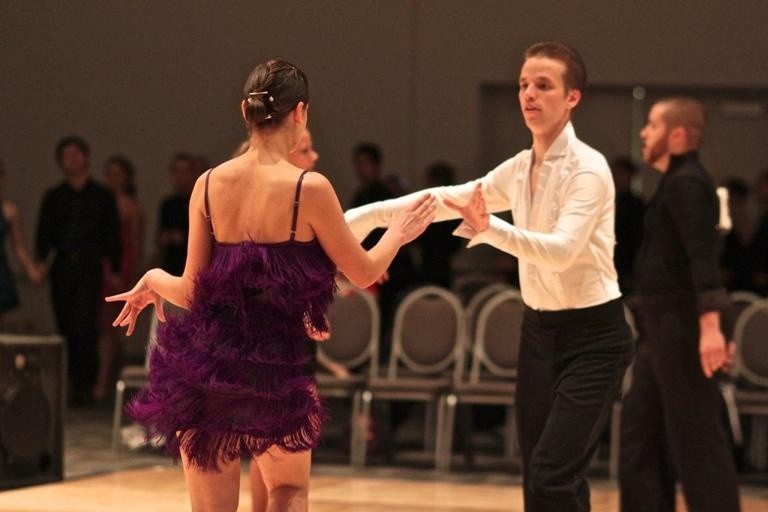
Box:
<box><xmin>105</xmin><ymin>60</ymin><xmax>435</xmax><ymax>512</ymax></box>
<box><xmin>348</xmin><ymin>141</ymin><xmax>520</xmax><ymax>450</ymax></box>
<box><xmin>609</xmin><ymin>154</ymin><xmax>768</xmax><ymax>300</ymax></box>
<box><xmin>2</xmin><ymin>136</ymin><xmax>209</xmax><ymax>408</ymax></box>
<box><xmin>617</xmin><ymin>97</ymin><xmax>742</xmax><ymax>512</ymax></box>
<box><xmin>344</xmin><ymin>44</ymin><xmax>623</xmax><ymax>512</ymax></box>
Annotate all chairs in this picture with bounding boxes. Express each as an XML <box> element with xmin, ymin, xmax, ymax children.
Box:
<box><xmin>444</xmin><ymin>291</ymin><xmax>524</xmax><ymax>476</ymax></box>
<box><xmin>604</xmin><ymin>303</ymin><xmax>640</xmax><ymax>481</ymax></box>
<box><xmin>108</xmin><ymin>303</ymin><xmax>165</xmax><ymax>457</ymax></box>
<box><xmin>715</xmin><ymin>293</ymin><xmax>762</xmax><ymax>407</ymax></box>
<box><xmin>362</xmin><ymin>287</ymin><xmax>465</xmax><ymax>472</ymax></box>
<box><xmin>462</xmin><ymin>283</ymin><xmax>515</xmax><ymax>383</ymax></box>
<box><xmin>719</xmin><ymin>300</ymin><xmax>764</xmax><ymax>480</ymax></box>
<box><xmin>312</xmin><ymin>285</ymin><xmax>381</xmax><ymax>465</ymax></box>
